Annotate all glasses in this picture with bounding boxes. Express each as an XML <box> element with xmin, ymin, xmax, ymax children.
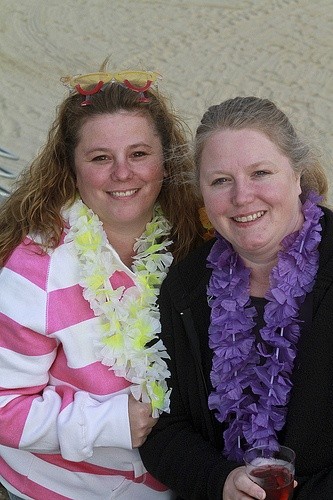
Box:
<box><xmin>67</xmin><ymin>70</ymin><xmax>156</xmax><ymax>108</ymax></box>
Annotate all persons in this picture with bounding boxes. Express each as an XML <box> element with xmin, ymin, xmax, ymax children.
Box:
<box><xmin>137</xmin><ymin>96</ymin><xmax>333</xmax><ymax>500</ymax></box>
<box><xmin>0</xmin><ymin>72</ymin><xmax>204</xmax><ymax>500</ymax></box>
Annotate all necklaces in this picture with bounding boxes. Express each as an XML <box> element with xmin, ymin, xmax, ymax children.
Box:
<box><xmin>203</xmin><ymin>186</ymin><xmax>324</xmax><ymax>466</ymax></box>
<box><xmin>58</xmin><ymin>187</ymin><xmax>175</xmax><ymax>419</ymax></box>
